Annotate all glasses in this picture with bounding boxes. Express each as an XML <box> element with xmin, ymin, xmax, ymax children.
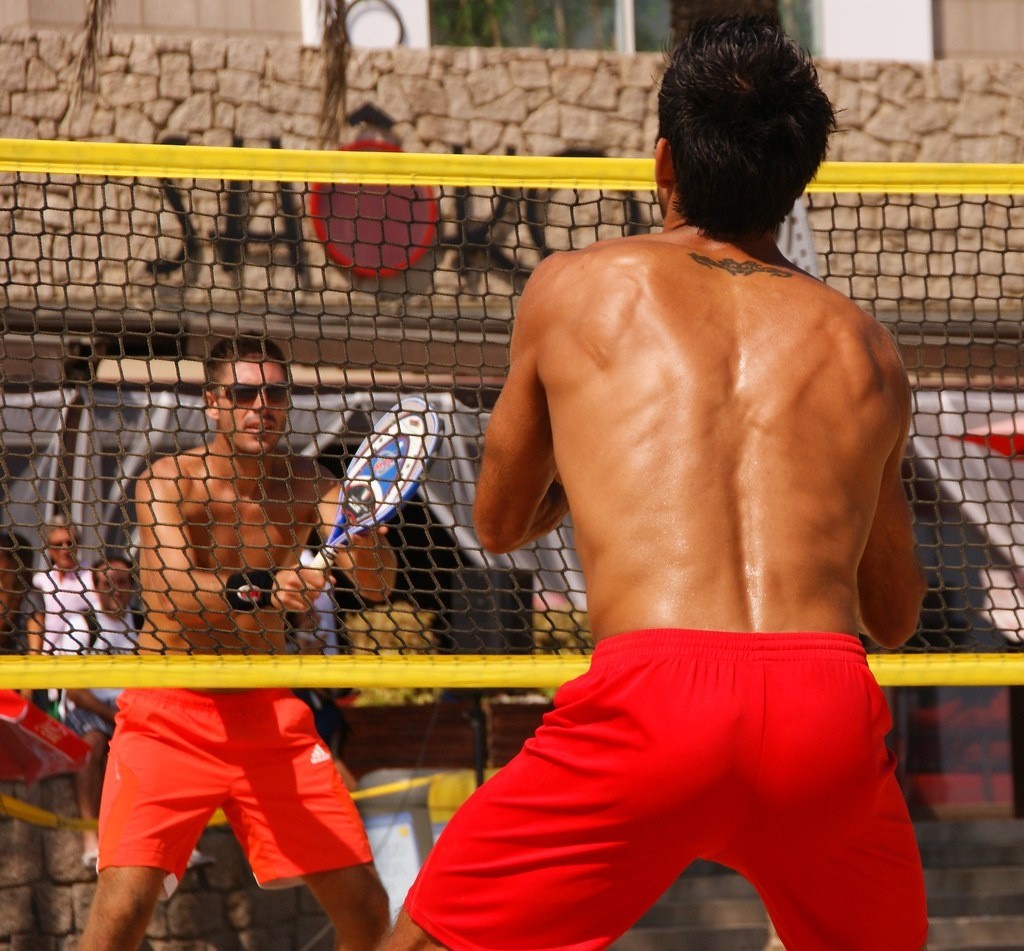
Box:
<box><xmin>208</xmin><ymin>381</ymin><xmax>290</xmax><ymax>405</ymax></box>
<box><xmin>48</xmin><ymin>538</ymin><xmax>77</xmax><ymax>551</ymax></box>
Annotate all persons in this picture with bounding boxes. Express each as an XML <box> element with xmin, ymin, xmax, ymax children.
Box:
<box><xmin>285</xmin><ymin>605</ymin><xmax>362</xmax><ymax>790</ymax></box>
<box><xmin>58</xmin><ymin>555</ymin><xmax>218</xmax><ymax>870</ymax></box>
<box><xmin>385</xmin><ymin>17</ymin><xmax>928</xmax><ymax>951</ymax></box>
<box><xmin>0</xmin><ymin>533</ymin><xmax>47</xmax><ymax>700</ymax></box>
<box><xmin>32</xmin><ymin>515</ymin><xmax>96</xmax><ymax>699</ymax></box>
<box><xmin>75</xmin><ymin>330</ymin><xmax>390</xmax><ymax>951</ymax></box>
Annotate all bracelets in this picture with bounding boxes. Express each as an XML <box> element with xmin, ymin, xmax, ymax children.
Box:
<box><xmin>225</xmin><ymin>567</ymin><xmax>283</xmax><ymax>612</ymax></box>
